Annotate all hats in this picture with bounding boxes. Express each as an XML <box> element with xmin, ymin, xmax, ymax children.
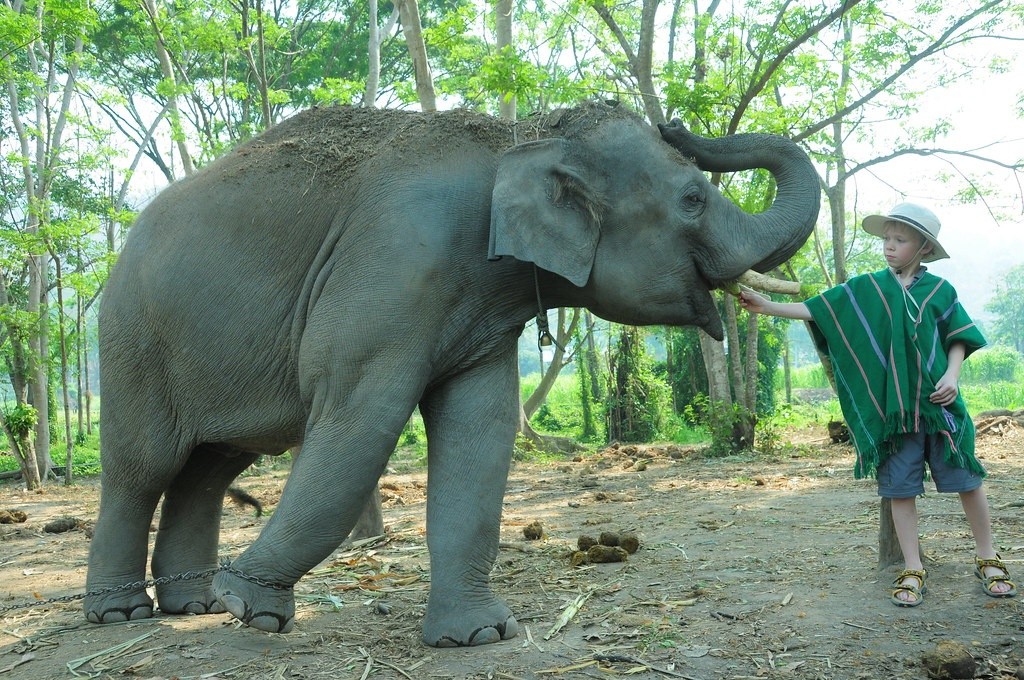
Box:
<box><xmin>862</xmin><ymin>203</ymin><xmax>950</xmax><ymax>263</ymax></box>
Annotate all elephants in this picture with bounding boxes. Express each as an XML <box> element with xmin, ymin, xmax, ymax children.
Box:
<box><xmin>81</xmin><ymin>103</ymin><xmax>822</xmax><ymax>644</ymax></box>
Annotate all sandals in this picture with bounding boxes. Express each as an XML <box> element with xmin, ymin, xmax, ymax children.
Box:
<box><xmin>974</xmin><ymin>552</ymin><xmax>1017</xmax><ymax>598</ymax></box>
<box><xmin>891</xmin><ymin>568</ymin><xmax>929</xmax><ymax>606</ymax></box>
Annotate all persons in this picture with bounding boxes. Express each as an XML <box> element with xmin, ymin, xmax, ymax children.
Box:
<box><xmin>738</xmin><ymin>201</ymin><xmax>1017</xmax><ymax>607</ymax></box>
